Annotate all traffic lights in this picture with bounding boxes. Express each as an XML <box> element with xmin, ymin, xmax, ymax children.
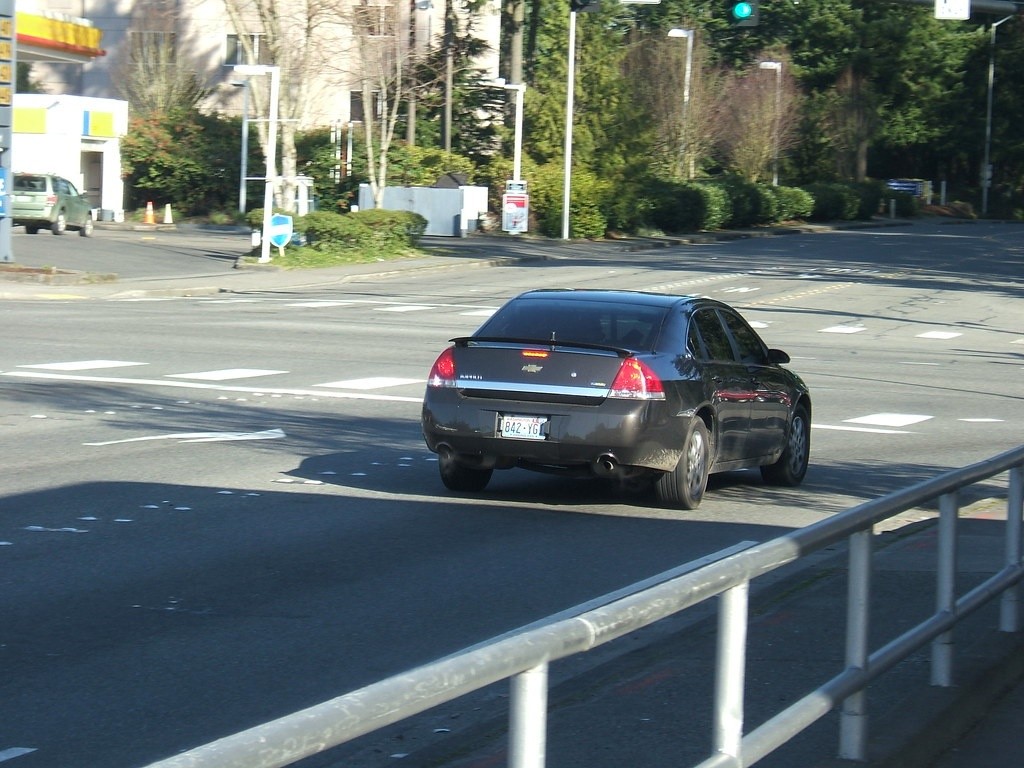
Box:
<box><xmin>730</xmin><ymin>1</ymin><xmax>758</xmax><ymax>28</ymax></box>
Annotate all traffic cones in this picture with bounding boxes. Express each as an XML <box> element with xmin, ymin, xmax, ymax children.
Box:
<box><xmin>162</xmin><ymin>204</ymin><xmax>174</xmax><ymax>225</ymax></box>
<box><xmin>144</xmin><ymin>202</ymin><xmax>156</xmax><ymax>225</ymax></box>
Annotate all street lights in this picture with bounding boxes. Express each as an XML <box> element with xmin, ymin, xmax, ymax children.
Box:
<box><xmin>234</xmin><ymin>64</ymin><xmax>281</xmax><ymax>262</ymax></box>
<box><xmin>759</xmin><ymin>61</ymin><xmax>782</xmax><ymax>187</ymax></box>
<box><xmin>981</xmin><ymin>14</ymin><xmax>1023</xmax><ymax>213</ymax></box>
<box><xmin>496</xmin><ymin>78</ymin><xmax>524</xmax><ymax>231</ymax></box>
<box><xmin>668</xmin><ymin>29</ymin><xmax>694</xmax><ymax>175</ymax></box>
<box><xmin>231</xmin><ymin>79</ymin><xmax>250</xmax><ymax>217</ymax></box>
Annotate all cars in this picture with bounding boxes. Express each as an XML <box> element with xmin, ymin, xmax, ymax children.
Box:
<box><xmin>420</xmin><ymin>285</ymin><xmax>812</xmax><ymax>511</ymax></box>
<box><xmin>12</xmin><ymin>173</ymin><xmax>94</xmax><ymax>237</ymax></box>
